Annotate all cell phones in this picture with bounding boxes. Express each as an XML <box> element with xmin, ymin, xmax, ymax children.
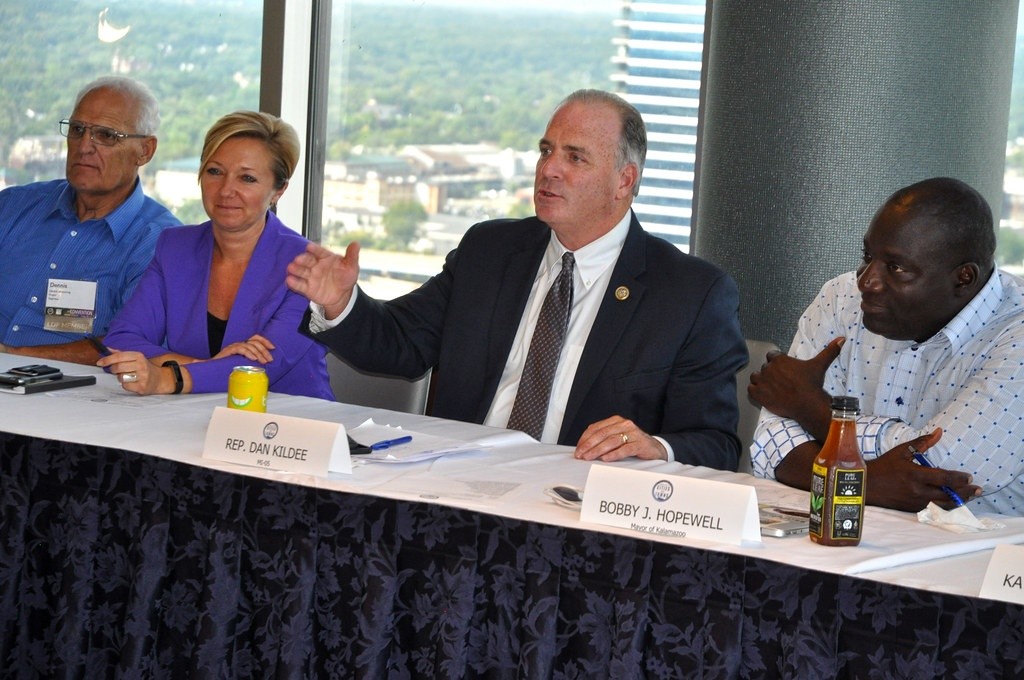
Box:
<box><xmin>0</xmin><ymin>365</ymin><xmax>64</xmax><ymax>384</ymax></box>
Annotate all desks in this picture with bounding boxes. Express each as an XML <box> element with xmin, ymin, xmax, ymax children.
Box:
<box><xmin>0</xmin><ymin>352</ymin><xmax>1024</xmax><ymax>680</ymax></box>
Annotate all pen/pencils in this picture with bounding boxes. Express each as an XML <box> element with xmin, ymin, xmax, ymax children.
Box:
<box><xmin>85</xmin><ymin>334</ymin><xmax>113</xmax><ymax>356</ymax></box>
<box><xmin>908</xmin><ymin>445</ymin><xmax>965</xmax><ymax>508</ymax></box>
<box><xmin>370</xmin><ymin>435</ymin><xmax>413</xmax><ymax>450</ymax></box>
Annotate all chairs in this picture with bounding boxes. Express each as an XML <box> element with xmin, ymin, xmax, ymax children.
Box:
<box><xmin>324</xmin><ymin>350</ymin><xmax>433</xmax><ymax>415</ymax></box>
<box><xmin>735</xmin><ymin>341</ymin><xmax>779</xmax><ymax>478</ymax></box>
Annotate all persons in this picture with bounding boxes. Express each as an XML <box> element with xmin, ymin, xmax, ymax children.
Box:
<box><xmin>96</xmin><ymin>112</ymin><xmax>337</xmax><ymax>401</ymax></box>
<box><xmin>283</xmin><ymin>91</ymin><xmax>749</xmax><ymax>472</ymax></box>
<box><xmin>747</xmin><ymin>177</ymin><xmax>1024</xmax><ymax>521</ymax></box>
<box><xmin>0</xmin><ymin>77</ymin><xmax>183</xmax><ymax>366</ymax></box>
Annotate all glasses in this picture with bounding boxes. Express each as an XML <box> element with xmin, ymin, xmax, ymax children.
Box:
<box><xmin>59</xmin><ymin>118</ymin><xmax>151</xmax><ymax>146</ymax></box>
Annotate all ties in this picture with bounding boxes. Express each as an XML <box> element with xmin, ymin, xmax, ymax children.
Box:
<box><xmin>506</xmin><ymin>253</ymin><xmax>575</xmax><ymax>442</ymax></box>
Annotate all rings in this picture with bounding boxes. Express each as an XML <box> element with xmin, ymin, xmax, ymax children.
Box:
<box><xmin>621</xmin><ymin>434</ymin><xmax>628</xmax><ymax>444</ymax></box>
<box><xmin>122</xmin><ymin>373</ymin><xmax>137</xmax><ymax>383</ymax></box>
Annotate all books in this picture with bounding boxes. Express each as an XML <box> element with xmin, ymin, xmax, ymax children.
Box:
<box><xmin>0</xmin><ymin>372</ymin><xmax>96</xmax><ymax>395</ymax></box>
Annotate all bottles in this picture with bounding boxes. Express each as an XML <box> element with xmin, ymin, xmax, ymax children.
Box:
<box><xmin>809</xmin><ymin>396</ymin><xmax>867</xmax><ymax>546</ymax></box>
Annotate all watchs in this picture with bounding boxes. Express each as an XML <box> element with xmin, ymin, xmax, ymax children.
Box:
<box><xmin>162</xmin><ymin>360</ymin><xmax>184</xmax><ymax>394</ymax></box>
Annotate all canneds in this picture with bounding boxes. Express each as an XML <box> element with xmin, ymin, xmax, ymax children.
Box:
<box><xmin>227</xmin><ymin>366</ymin><xmax>268</xmax><ymax>412</ymax></box>
